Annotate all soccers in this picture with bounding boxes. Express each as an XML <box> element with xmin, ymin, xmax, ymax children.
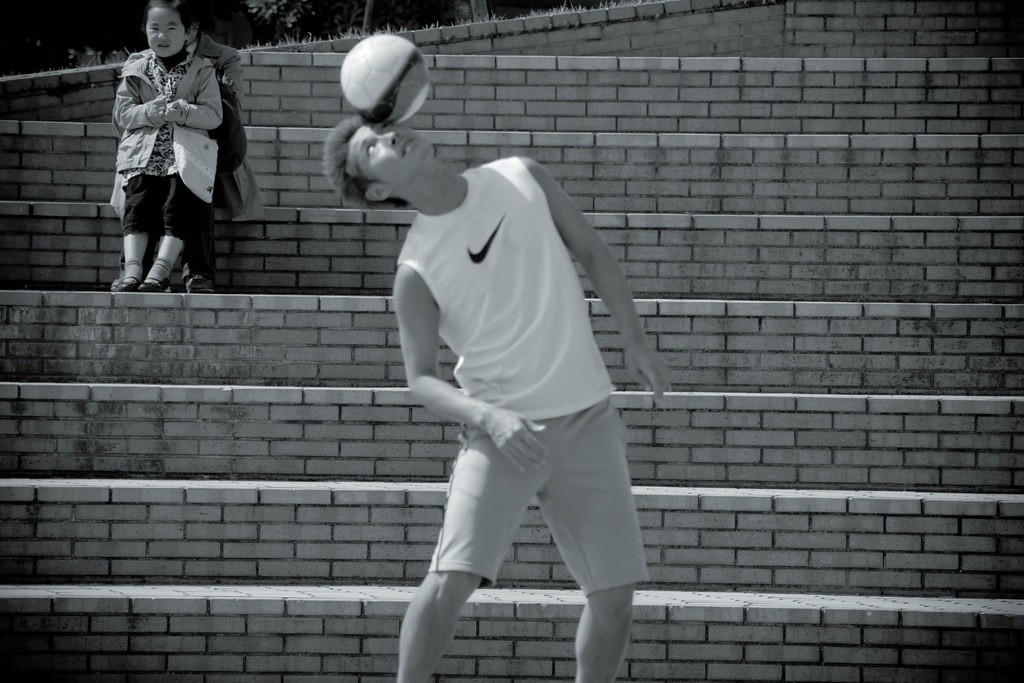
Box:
<box><xmin>340</xmin><ymin>34</ymin><xmax>431</xmax><ymax>125</ymax></box>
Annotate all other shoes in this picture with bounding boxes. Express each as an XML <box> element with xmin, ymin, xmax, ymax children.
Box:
<box><xmin>115</xmin><ymin>276</ymin><xmax>140</xmax><ymax>291</ymax></box>
<box><xmin>185</xmin><ymin>274</ymin><xmax>214</xmax><ymax>293</ymax></box>
<box><xmin>138</xmin><ymin>276</ymin><xmax>171</xmax><ymax>291</ymax></box>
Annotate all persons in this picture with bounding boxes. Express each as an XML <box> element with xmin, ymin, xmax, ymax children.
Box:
<box><xmin>109</xmin><ymin>0</ymin><xmax>223</xmax><ymax>292</ymax></box>
<box><xmin>323</xmin><ymin>114</ymin><xmax>670</xmax><ymax>683</ymax></box>
<box><xmin>112</xmin><ymin>0</ymin><xmax>248</xmax><ymax>292</ymax></box>
